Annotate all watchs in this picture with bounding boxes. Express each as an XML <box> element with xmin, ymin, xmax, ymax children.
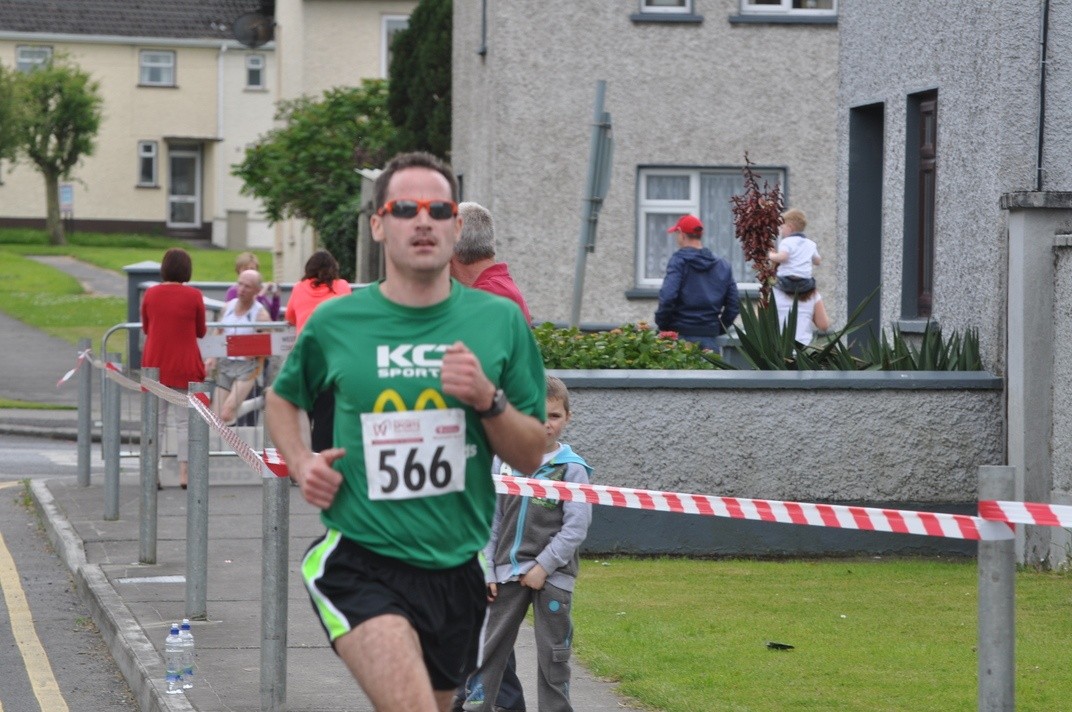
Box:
<box><xmin>479</xmin><ymin>387</ymin><xmax>507</xmax><ymax>420</ymax></box>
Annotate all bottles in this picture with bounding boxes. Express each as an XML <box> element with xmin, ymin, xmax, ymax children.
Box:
<box><xmin>164</xmin><ymin>619</ymin><xmax>195</xmax><ymax>695</ymax></box>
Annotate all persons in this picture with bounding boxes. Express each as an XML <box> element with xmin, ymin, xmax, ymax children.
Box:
<box><xmin>202</xmin><ymin>270</ymin><xmax>273</xmax><ymax>425</ymax></box>
<box><xmin>225</xmin><ymin>253</ymin><xmax>281</xmax><ymax>427</ymax></box>
<box><xmin>266</xmin><ymin>151</ymin><xmax>547</xmax><ymax>712</ymax></box>
<box><xmin>767</xmin><ymin>207</ymin><xmax>821</xmax><ymax>297</ymax></box>
<box><xmin>447</xmin><ymin>201</ymin><xmax>533</xmax><ymax>328</ymax></box>
<box><xmin>654</xmin><ymin>214</ymin><xmax>740</xmax><ymax>362</ymax></box>
<box><xmin>285</xmin><ymin>250</ymin><xmax>353</xmax><ymax>344</ymax></box>
<box><xmin>459</xmin><ymin>374</ymin><xmax>593</xmax><ymax>712</ymax></box>
<box><xmin>756</xmin><ymin>284</ymin><xmax>831</xmax><ymax>356</ymax></box>
<box><xmin>139</xmin><ymin>247</ymin><xmax>216</xmax><ymax>492</ymax></box>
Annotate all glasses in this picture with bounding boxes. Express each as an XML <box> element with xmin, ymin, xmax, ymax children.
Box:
<box><xmin>377</xmin><ymin>197</ymin><xmax>458</xmax><ymax>222</ymax></box>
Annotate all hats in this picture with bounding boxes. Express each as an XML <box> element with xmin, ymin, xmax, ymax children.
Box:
<box><xmin>667</xmin><ymin>215</ymin><xmax>703</xmax><ymax>235</ymax></box>
